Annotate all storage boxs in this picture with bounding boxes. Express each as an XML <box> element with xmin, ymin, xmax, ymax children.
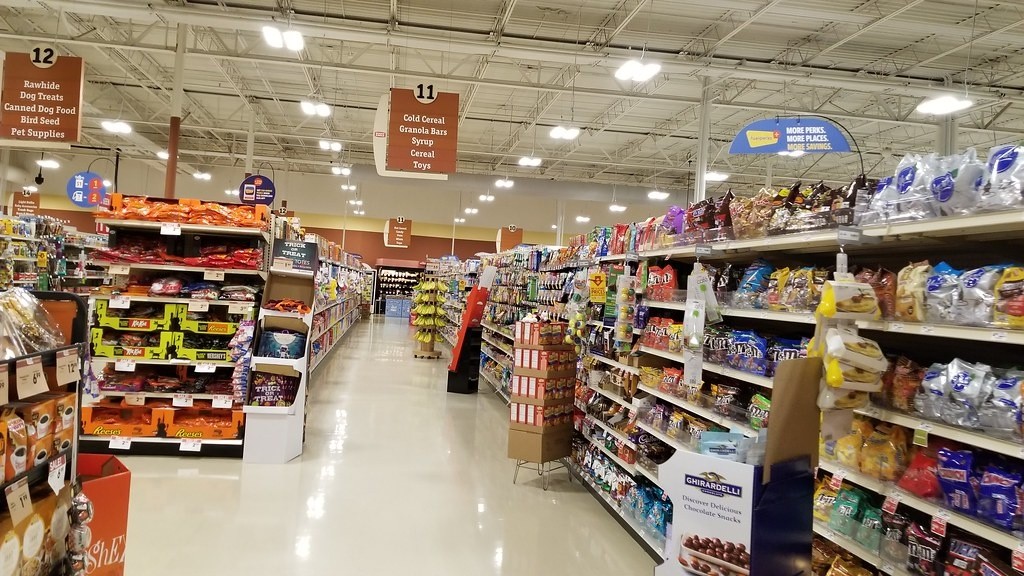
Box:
<box><xmin>510</xmin><ymin>319</ymin><xmax>577</xmax><ymax>429</ymax></box>
<box><xmin>0</xmin><ymin>391</ymin><xmax>79</xmax><ymax>486</ymax></box>
<box><xmin>78</xmin><ymin>454</ymin><xmax>130</xmax><ymax>576</ymax></box>
<box><xmin>305</xmin><ymin>232</ymin><xmax>342</xmax><ymax>261</ymax></box>
<box><xmin>32</xmin><ymin>297</ymin><xmax>76</xmax><ymax>348</ymax></box>
<box><xmin>665</xmin><ymin>356</ymin><xmax>825</xmax><ymax>576</ymax></box>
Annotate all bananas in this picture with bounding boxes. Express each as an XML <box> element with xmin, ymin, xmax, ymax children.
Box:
<box><xmin>412</xmin><ymin>281</ymin><xmax>448</xmax><ymax>343</ymax></box>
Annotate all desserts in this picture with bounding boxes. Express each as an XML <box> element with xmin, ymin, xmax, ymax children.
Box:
<box><xmin>679</xmin><ymin>535</ymin><xmax>751</xmax><ymax>576</ymax></box>
<box><xmin>836</xmin><ymin>287</ymin><xmax>883</xmax><ymax>408</ymax></box>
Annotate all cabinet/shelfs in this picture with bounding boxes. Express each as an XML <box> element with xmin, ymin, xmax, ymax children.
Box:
<box><xmin>0</xmin><ymin>219</ymin><xmax>109</xmax><ymax>296</ymax></box>
<box><xmin>78</xmin><ymin>214</ymin><xmax>273</xmax><ymax>447</ymax></box>
<box><xmin>0</xmin><ymin>289</ymin><xmax>89</xmax><ymax>576</ymax></box>
<box><xmin>506</xmin><ymin>321</ymin><xmax>577</xmax><ymax>490</ymax></box>
<box><xmin>306</xmin><ymin>255</ymin><xmax>540</xmax><ymax>407</ymax></box>
<box><xmin>541</xmin><ymin>210</ymin><xmax>1024</xmax><ymax>576</ymax></box>
